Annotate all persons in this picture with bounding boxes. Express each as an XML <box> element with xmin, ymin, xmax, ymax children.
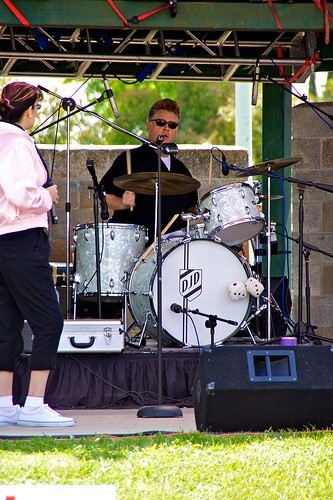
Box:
<box><xmin>0</xmin><ymin>82</ymin><xmax>75</xmax><ymax>426</ymax></box>
<box><xmin>99</xmin><ymin>98</ymin><xmax>199</xmax><ymax>319</ymax></box>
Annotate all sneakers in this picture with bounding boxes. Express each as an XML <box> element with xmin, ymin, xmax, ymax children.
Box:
<box><xmin>0</xmin><ymin>404</ymin><xmax>22</xmax><ymax>427</ymax></box>
<box><xmin>17</xmin><ymin>403</ymin><xmax>75</xmax><ymax>427</ymax></box>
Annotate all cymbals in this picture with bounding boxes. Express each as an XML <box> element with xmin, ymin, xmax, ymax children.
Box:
<box><xmin>235</xmin><ymin>156</ymin><xmax>304</xmax><ymax>178</ymax></box>
<box><xmin>258</xmin><ymin>194</ymin><xmax>285</xmax><ymax>199</ymax></box>
<box><xmin>113</xmin><ymin>171</ymin><xmax>201</xmax><ymax>195</ymax></box>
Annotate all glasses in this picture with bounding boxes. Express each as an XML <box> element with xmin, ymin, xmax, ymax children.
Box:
<box><xmin>149</xmin><ymin>119</ymin><xmax>179</xmax><ymax>130</ymax></box>
<box><xmin>26</xmin><ymin>103</ymin><xmax>41</xmax><ymax>110</ymax></box>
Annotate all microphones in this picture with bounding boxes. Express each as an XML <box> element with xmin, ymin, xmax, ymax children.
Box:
<box><xmin>222</xmin><ymin>154</ymin><xmax>229</xmax><ymax>176</ymax></box>
<box><xmin>46</xmin><ymin>178</ymin><xmax>59</xmax><ymax>225</ymax></box>
<box><xmin>252</xmin><ymin>64</ymin><xmax>260</xmax><ymax>106</ymax></box>
<box><xmin>100</xmin><ymin>185</ymin><xmax>109</xmax><ymax>220</ymax></box>
<box><xmin>161</xmin><ymin>143</ymin><xmax>179</xmax><ymax>154</ymax></box>
<box><xmin>103</xmin><ymin>79</ymin><xmax>120</xmax><ymax>119</ymax></box>
<box><xmin>170</xmin><ymin>303</ymin><xmax>182</xmax><ymax>313</ymax></box>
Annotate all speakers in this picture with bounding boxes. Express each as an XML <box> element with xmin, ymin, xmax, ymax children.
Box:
<box><xmin>192</xmin><ymin>344</ymin><xmax>333</xmax><ymax>432</ymax></box>
<box><xmin>233</xmin><ymin>276</ymin><xmax>292</xmax><ymax>338</ymax></box>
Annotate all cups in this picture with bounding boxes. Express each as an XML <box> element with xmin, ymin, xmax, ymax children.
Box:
<box><xmin>280</xmin><ymin>337</ymin><xmax>298</xmax><ymax>345</ymax></box>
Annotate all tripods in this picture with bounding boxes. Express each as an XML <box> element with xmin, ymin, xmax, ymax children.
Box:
<box><xmin>240</xmin><ymin>167</ymin><xmax>333</xmax><ymax>343</ymax></box>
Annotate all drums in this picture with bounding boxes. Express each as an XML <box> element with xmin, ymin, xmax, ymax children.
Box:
<box><xmin>72</xmin><ymin>222</ymin><xmax>149</xmax><ymax>303</ymax></box>
<box><xmin>159</xmin><ymin>228</ymin><xmax>200</xmax><ymax>243</ymax></box>
<box><xmin>196</xmin><ymin>182</ymin><xmax>266</xmax><ymax>247</ymax></box>
<box><xmin>128</xmin><ymin>236</ymin><xmax>255</xmax><ymax>348</ymax></box>
